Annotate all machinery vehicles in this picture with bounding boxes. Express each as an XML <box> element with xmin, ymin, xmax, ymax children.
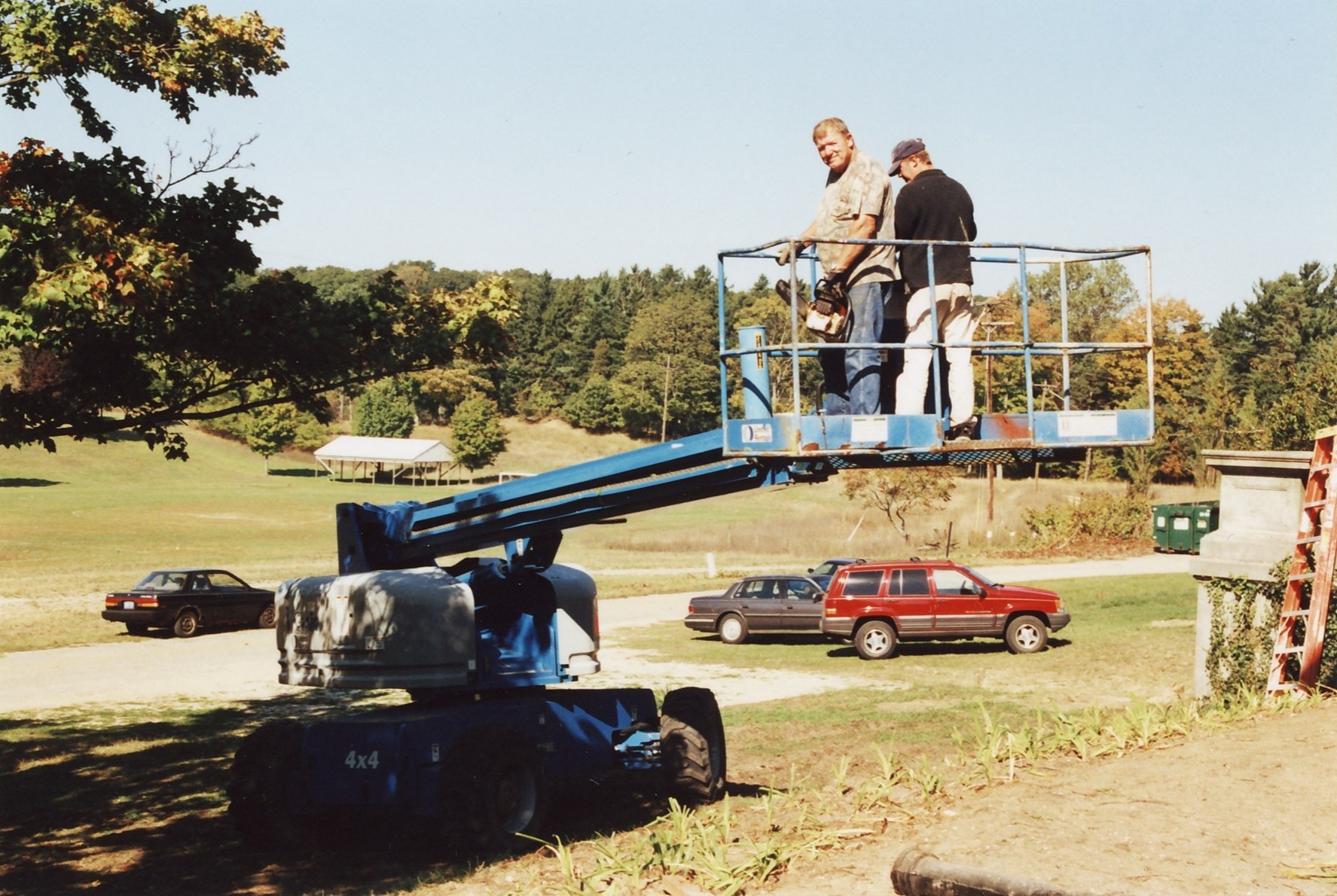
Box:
<box><xmin>226</xmin><ymin>236</ymin><xmax>1160</xmax><ymax>857</ymax></box>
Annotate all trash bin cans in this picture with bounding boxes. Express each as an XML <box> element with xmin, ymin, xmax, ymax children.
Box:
<box><xmin>1150</xmin><ymin>501</ymin><xmax>1221</xmax><ymax>554</ymax></box>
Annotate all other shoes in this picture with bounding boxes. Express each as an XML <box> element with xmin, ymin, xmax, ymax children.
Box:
<box><xmin>943</xmin><ymin>423</ymin><xmax>976</xmax><ymax>442</ymax></box>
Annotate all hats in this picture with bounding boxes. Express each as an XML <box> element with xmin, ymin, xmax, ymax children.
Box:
<box><xmin>888</xmin><ymin>138</ymin><xmax>925</xmax><ymax>176</ymax></box>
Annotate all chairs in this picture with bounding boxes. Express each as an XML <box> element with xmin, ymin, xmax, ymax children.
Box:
<box><xmin>787</xmin><ymin>589</ymin><xmax>800</xmax><ymax>599</ymax></box>
<box><xmin>195</xmin><ymin>577</ymin><xmax>206</xmax><ymax>590</ymax></box>
<box><xmin>173</xmin><ymin>577</ymin><xmax>185</xmax><ymax>588</ymax></box>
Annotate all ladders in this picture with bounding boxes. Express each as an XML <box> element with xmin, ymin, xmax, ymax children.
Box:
<box><xmin>1266</xmin><ymin>424</ymin><xmax>1337</xmax><ymax>697</ymax></box>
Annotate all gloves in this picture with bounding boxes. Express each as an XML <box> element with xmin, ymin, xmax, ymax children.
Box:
<box><xmin>826</xmin><ymin>270</ymin><xmax>846</xmax><ymax>290</ymax></box>
<box><xmin>777</xmin><ymin>242</ymin><xmax>806</xmax><ymax>265</ymax></box>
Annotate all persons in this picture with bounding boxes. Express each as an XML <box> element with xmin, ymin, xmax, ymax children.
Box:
<box><xmin>888</xmin><ymin>138</ymin><xmax>977</xmax><ymax>416</ymax></box>
<box><xmin>775</xmin><ymin>117</ymin><xmax>901</xmax><ymax>416</ymax></box>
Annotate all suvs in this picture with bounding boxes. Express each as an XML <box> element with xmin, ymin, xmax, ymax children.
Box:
<box><xmin>820</xmin><ymin>556</ymin><xmax>1072</xmax><ymax>660</ymax></box>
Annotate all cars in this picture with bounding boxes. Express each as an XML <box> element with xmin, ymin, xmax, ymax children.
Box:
<box><xmin>102</xmin><ymin>569</ymin><xmax>276</xmax><ymax>637</ymax></box>
<box><xmin>788</xmin><ymin>558</ymin><xmax>873</xmax><ymax>600</ymax></box>
<box><xmin>683</xmin><ymin>573</ymin><xmax>856</xmax><ymax>645</ymax></box>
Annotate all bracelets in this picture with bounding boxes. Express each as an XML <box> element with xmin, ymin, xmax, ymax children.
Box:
<box><xmin>830</xmin><ymin>264</ymin><xmax>845</xmax><ymax>272</ymax></box>
